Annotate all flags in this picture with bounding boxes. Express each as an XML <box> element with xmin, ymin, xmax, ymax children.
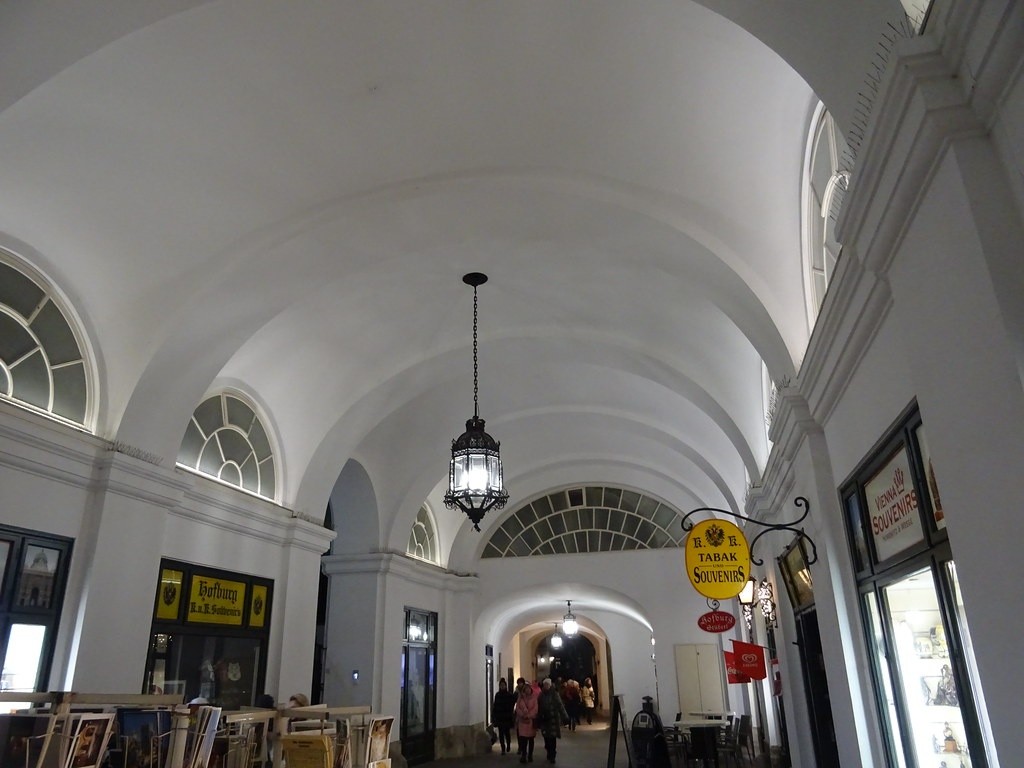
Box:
<box><xmin>724</xmin><ymin>651</ymin><xmax>752</xmax><ymax>684</ymax></box>
<box><xmin>733</xmin><ymin>639</ymin><xmax>767</xmax><ymax>680</ymax></box>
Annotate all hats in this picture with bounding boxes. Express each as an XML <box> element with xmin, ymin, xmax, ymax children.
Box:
<box><xmin>499</xmin><ymin>678</ymin><xmax>506</xmax><ymax>686</ymax></box>
<box><xmin>543</xmin><ymin>678</ymin><xmax>552</xmax><ymax>686</ymax></box>
<box><xmin>574</xmin><ymin>681</ymin><xmax>579</xmax><ymax>687</ymax></box>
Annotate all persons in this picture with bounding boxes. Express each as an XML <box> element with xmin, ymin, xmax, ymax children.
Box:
<box><xmin>490</xmin><ymin>678</ymin><xmax>513</xmax><ymax>756</ymax></box>
<box><xmin>512</xmin><ymin>677</ymin><xmax>529</xmax><ymax>755</ymax></box>
<box><xmin>254</xmin><ymin>692</ymin><xmax>276</xmax><ymax>768</ymax></box>
<box><xmin>538</xmin><ymin>678</ymin><xmax>570</xmax><ymax>763</ymax></box>
<box><xmin>287</xmin><ymin>693</ymin><xmax>310</xmax><ymax>734</ymax></box>
<box><xmin>515</xmin><ymin>684</ymin><xmax>540</xmax><ymax>763</ymax></box>
<box><xmin>542</xmin><ymin>675</ymin><xmax>580</xmax><ymax>733</ymax></box>
<box><xmin>581</xmin><ymin>677</ymin><xmax>595</xmax><ymax>725</ymax></box>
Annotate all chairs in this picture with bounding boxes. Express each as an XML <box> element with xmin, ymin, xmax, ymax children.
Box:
<box><xmin>662</xmin><ymin>712</ymin><xmax>755</xmax><ymax>768</ymax></box>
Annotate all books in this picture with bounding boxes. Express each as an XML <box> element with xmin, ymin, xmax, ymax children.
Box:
<box><xmin>2</xmin><ymin>704</ymin><xmax>396</xmax><ymax>768</ymax></box>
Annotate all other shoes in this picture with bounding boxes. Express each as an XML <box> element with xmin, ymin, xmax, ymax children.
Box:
<box><xmin>546</xmin><ymin>755</ymin><xmax>556</xmax><ymax>764</ymax></box>
<box><xmin>517</xmin><ymin>750</ymin><xmax>522</xmax><ymax>755</ymax></box>
<box><xmin>519</xmin><ymin>757</ymin><xmax>533</xmax><ymax>764</ymax></box>
<box><xmin>500</xmin><ymin>745</ymin><xmax>510</xmax><ymax>756</ymax></box>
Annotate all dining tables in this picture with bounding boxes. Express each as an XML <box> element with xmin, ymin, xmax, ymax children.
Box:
<box><xmin>674</xmin><ymin>719</ymin><xmax>731</xmax><ymax>758</ymax></box>
<box><xmin>688</xmin><ymin>712</ymin><xmax>737</xmax><ymax>744</ymax></box>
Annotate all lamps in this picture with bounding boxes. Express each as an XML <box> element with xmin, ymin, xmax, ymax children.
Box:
<box><xmin>443</xmin><ymin>272</ymin><xmax>512</xmax><ymax>533</ymax></box>
<box><xmin>551</xmin><ymin>623</ymin><xmax>563</xmax><ymax>650</ymax></box>
<box><xmin>562</xmin><ymin>600</ymin><xmax>579</xmax><ymax>639</ymax></box>
<box><xmin>738</xmin><ymin>576</ymin><xmax>779</xmax><ymax>629</ymax></box>
